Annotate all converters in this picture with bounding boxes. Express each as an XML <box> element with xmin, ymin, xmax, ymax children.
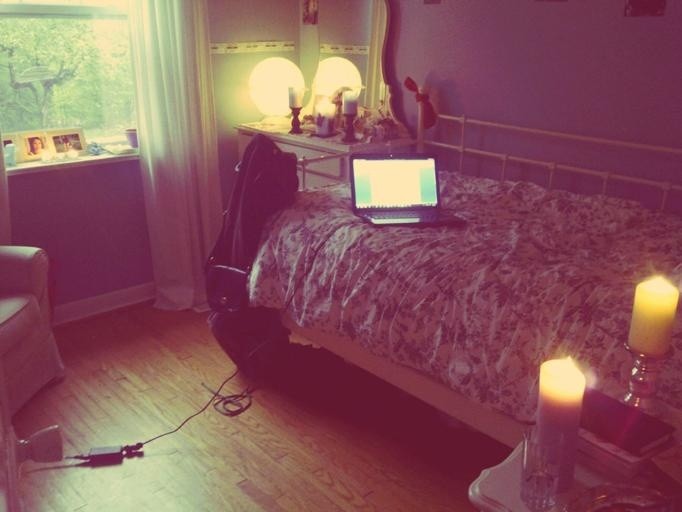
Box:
<box><xmin>88</xmin><ymin>446</ymin><xmax>123</xmax><ymax>464</ymax></box>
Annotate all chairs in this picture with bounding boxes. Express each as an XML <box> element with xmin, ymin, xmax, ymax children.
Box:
<box><xmin>0</xmin><ymin>246</ymin><xmax>65</xmax><ymax>420</ymax></box>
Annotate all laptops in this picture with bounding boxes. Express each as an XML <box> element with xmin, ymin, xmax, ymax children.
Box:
<box><xmin>349</xmin><ymin>152</ymin><xmax>467</xmax><ymax>227</ymax></box>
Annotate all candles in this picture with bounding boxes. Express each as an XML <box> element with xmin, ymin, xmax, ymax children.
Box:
<box><xmin>289</xmin><ymin>87</ymin><xmax>303</xmax><ymax>108</ymax></box>
<box><xmin>343</xmin><ymin>91</ymin><xmax>357</xmax><ymax>116</ymax></box>
<box><xmin>628</xmin><ymin>276</ymin><xmax>679</xmax><ymax>354</ymax></box>
<box><xmin>538</xmin><ymin>356</ymin><xmax>586</xmax><ymax>442</ymax></box>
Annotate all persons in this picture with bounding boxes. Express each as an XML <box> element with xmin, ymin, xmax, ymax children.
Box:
<box><xmin>29</xmin><ymin>136</ymin><xmax>44</xmax><ymax>157</ymax></box>
<box><xmin>61</xmin><ymin>135</ymin><xmax>73</xmax><ymax>151</ymax></box>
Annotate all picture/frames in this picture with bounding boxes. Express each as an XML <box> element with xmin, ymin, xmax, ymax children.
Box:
<box><xmin>1</xmin><ymin>127</ymin><xmax>87</xmax><ymax>164</ymax></box>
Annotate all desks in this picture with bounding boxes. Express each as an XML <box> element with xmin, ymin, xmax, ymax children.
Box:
<box><xmin>234</xmin><ymin>121</ymin><xmax>411</xmax><ymax>188</ymax></box>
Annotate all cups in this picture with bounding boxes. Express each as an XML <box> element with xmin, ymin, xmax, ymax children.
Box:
<box><xmin>6</xmin><ymin>144</ymin><xmax>16</xmax><ymax>166</ymax></box>
<box><xmin>125</xmin><ymin>128</ymin><xmax>138</xmax><ymax>148</ymax></box>
<box><xmin>520</xmin><ymin>424</ymin><xmax>564</xmax><ymax>512</ymax></box>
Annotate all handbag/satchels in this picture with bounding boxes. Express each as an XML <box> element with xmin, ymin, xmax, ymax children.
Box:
<box><xmin>207</xmin><ymin>308</ymin><xmax>289</xmax><ymax>371</ymax></box>
<box><xmin>203</xmin><ymin>258</ymin><xmax>249</xmax><ymax>314</ymax></box>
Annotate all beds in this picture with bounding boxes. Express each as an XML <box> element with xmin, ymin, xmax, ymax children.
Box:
<box><xmin>204</xmin><ymin>77</ymin><xmax>682</xmax><ymax>450</ymax></box>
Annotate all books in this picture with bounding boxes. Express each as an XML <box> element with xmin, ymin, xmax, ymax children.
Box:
<box><xmin>578</xmin><ymin>385</ymin><xmax>682</xmax><ymax>490</ymax></box>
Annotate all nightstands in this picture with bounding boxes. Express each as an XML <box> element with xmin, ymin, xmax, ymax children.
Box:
<box><xmin>469</xmin><ymin>425</ymin><xmax>603</xmax><ymax>512</ymax></box>
